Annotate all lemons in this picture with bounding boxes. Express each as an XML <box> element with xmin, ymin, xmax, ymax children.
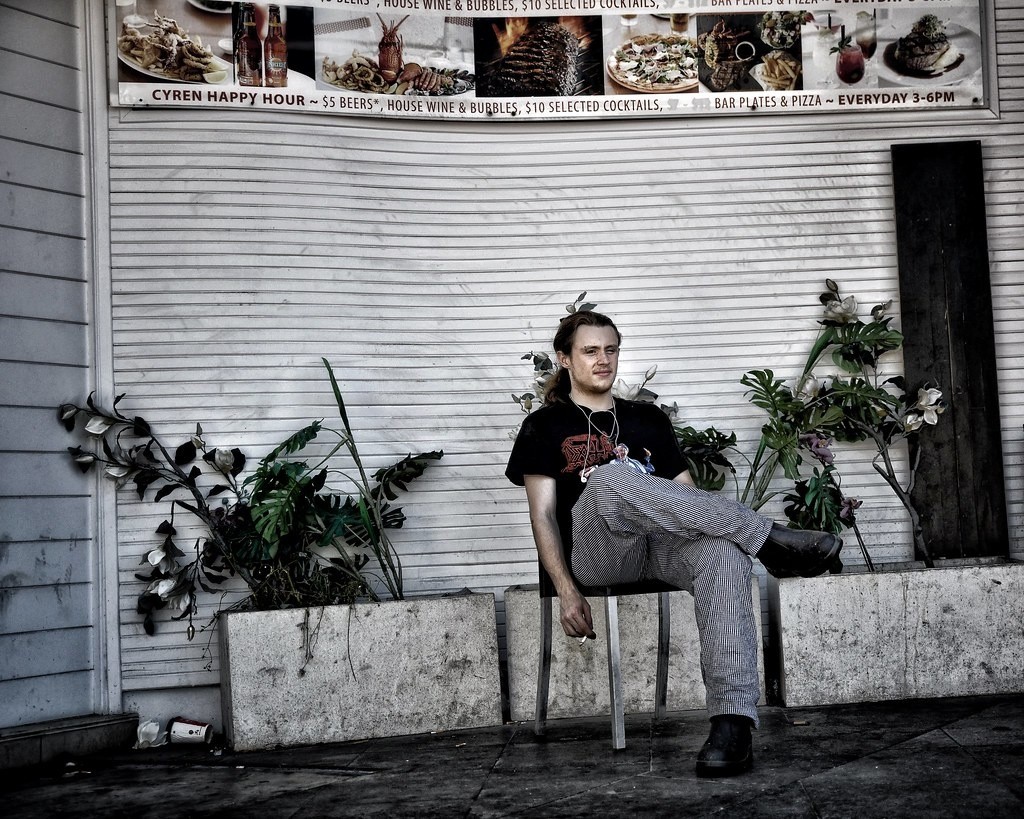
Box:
<box><xmin>201</xmin><ymin>71</ymin><xmax>228</xmax><ymax>84</ymax></box>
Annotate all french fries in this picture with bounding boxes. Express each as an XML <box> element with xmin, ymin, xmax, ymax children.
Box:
<box><xmin>759</xmin><ymin>50</ymin><xmax>803</xmax><ymax>91</ymax></box>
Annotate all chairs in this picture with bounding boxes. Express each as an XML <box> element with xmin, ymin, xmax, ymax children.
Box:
<box><xmin>534</xmin><ymin>555</ymin><xmax>685</xmax><ymax>752</ymax></box>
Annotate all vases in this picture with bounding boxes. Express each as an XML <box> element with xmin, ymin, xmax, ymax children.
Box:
<box><xmin>766</xmin><ymin>554</ymin><xmax>1024</xmax><ymax>708</ymax></box>
<box><xmin>213</xmin><ymin>588</ymin><xmax>504</xmax><ymax>755</ymax></box>
<box><xmin>501</xmin><ymin>575</ymin><xmax>769</xmax><ymax>722</ymax></box>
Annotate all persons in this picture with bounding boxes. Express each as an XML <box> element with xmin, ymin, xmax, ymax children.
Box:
<box><xmin>502</xmin><ymin>309</ymin><xmax>843</xmax><ymax>776</ymax></box>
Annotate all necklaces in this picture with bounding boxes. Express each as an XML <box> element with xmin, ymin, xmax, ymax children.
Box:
<box><xmin>566</xmin><ymin>391</ymin><xmax>617</xmax><ymax>445</ymax></box>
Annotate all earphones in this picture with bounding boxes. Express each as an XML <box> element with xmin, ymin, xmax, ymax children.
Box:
<box><xmin>581</xmin><ymin>469</ymin><xmax>587</xmax><ymax>483</ymax></box>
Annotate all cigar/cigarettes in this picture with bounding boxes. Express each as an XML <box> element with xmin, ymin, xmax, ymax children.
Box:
<box><xmin>578</xmin><ymin>634</ymin><xmax>588</xmax><ymax>646</ymax></box>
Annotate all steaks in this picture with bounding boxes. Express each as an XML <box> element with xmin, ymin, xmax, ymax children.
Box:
<box><xmin>895</xmin><ymin>31</ymin><xmax>950</xmax><ymax>68</ymax></box>
<box><xmin>709</xmin><ymin>61</ymin><xmax>747</xmax><ymax>90</ymax></box>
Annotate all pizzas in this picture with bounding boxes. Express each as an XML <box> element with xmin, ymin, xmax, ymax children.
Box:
<box><xmin>608</xmin><ymin>34</ymin><xmax>698</xmax><ymax>90</ymax></box>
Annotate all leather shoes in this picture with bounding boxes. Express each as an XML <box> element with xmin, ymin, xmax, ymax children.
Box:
<box><xmin>756</xmin><ymin>522</ymin><xmax>844</xmax><ymax>579</ymax></box>
<box><xmin>696</xmin><ymin>717</ymin><xmax>754</xmax><ymax>778</ymax></box>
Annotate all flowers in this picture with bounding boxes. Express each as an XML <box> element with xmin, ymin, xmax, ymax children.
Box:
<box><xmin>58</xmin><ymin>355</ymin><xmax>443</xmax><ymax>641</ymax></box>
<box><xmin>793</xmin><ymin>277</ymin><xmax>950</xmax><ymax>572</ymax></box>
<box><xmin>512</xmin><ymin>274</ymin><xmax>905</xmax><ymax>572</ymax></box>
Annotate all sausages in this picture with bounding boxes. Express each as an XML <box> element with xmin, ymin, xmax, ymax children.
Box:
<box><xmin>413</xmin><ymin>69</ymin><xmax>446</xmax><ymax>92</ymax></box>
